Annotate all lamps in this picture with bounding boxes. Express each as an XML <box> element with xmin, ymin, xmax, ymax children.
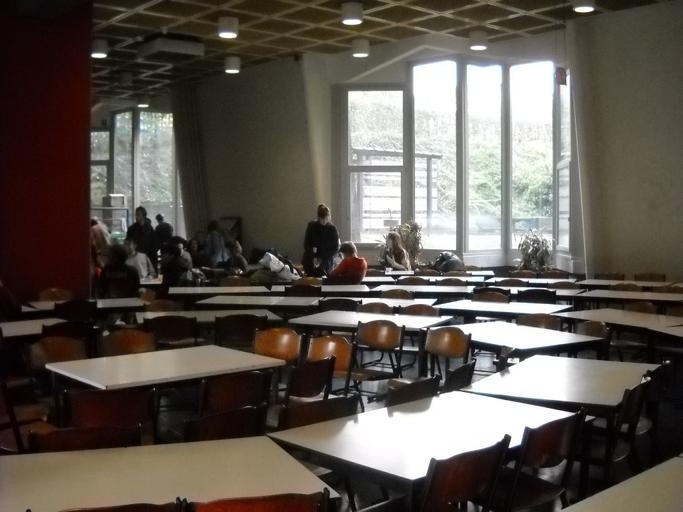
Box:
<box><xmin>571</xmin><ymin>2</ymin><xmax>596</xmax><ymax>17</ymax></box>
<box><xmin>349</xmin><ymin>36</ymin><xmax>374</xmax><ymax>59</ymax></box>
<box><xmin>467</xmin><ymin>27</ymin><xmax>492</xmax><ymax>54</ymax></box>
<box><xmin>340</xmin><ymin>2</ymin><xmax>366</xmax><ymax>26</ymax></box>
<box><xmin>223</xmin><ymin>56</ymin><xmax>241</xmax><ymax>73</ymax></box>
<box><xmin>216</xmin><ymin>16</ymin><xmax>239</xmax><ymax>41</ymax></box>
<box><xmin>89</xmin><ymin>38</ymin><xmax>112</xmax><ymax>60</ymax></box>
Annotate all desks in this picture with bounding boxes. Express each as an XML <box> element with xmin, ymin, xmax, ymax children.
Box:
<box><xmin>0</xmin><ymin>264</ymin><xmax>681</xmax><ymax>512</ymax></box>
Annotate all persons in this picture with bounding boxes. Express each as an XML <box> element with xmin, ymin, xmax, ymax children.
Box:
<box><xmin>376</xmin><ymin>231</ymin><xmax>411</xmax><ymax>280</ymax></box>
<box><xmin>327</xmin><ymin>240</ymin><xmax>368</xmax><ymax>285</ymax></box>
<box><xmin>301</xmin><ymin>203</ymin><xmax>340</xmax><ymax>278</ymax></box>
<box><xmin>90</xmin><ymin>205</ymin><xmax>250</xmax><ymax>335</ymax></box>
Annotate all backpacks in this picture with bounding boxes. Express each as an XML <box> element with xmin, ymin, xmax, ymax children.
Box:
<box><xmin>301</xmin><ymin>246</ymin><xmax>330</xmax><ymax>277</ymax></box>
